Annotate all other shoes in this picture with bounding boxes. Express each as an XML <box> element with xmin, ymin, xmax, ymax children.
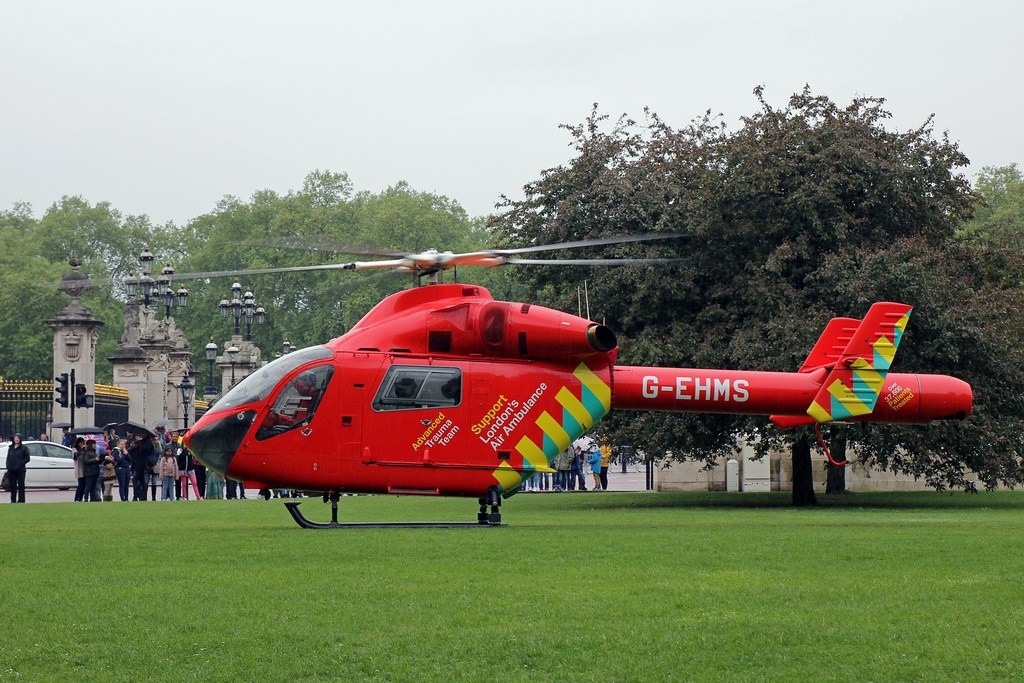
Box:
<box><xmin>579</xmin><ymin>487</ymin><xmax>587</xmax><ymax>491</ymax></box>
<box><xmin>593</xmin><ymin>488</ymin><xmax>600</xmax><ymax>491</ymax></box>
<box><xmin>528</xmin><ymin>487</ymin><xmax>532</xmax><ymax>491</ymax></box>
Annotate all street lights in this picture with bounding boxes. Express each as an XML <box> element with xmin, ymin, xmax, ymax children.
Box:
<box><xmin>218</xmin><ymin>277</ymin><xmax>267</xmax><ymax>343</ymax></box>
<box><xmin>178</xmin><ymin>372</ymin><xmax>195</xmax><ymax>429</ymax></box>
<box><xmin>126</xmin><ymin>249</ymin><xmax>189</xmax><ymax>320</ymax></box>
<box><xmin>225</xmin><ymin>343</ymin><xmax>240</xmax><ymax>386</ymax></box>
<box><xmin>202</xmin><ymin>335</ymin><xmax>220</xmax><ymax>400</ymax></box>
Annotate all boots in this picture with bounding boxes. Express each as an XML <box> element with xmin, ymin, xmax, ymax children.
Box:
<box><xmin>103</xmin><ymin>496</ymin><xmax>112</xmax><ymax>501</ymax></box>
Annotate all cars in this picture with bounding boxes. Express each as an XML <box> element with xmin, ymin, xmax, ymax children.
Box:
<box><xmin>0</xmin><ymin>440</ymin><xmax>79</xmax><ymax>490</ymax></box>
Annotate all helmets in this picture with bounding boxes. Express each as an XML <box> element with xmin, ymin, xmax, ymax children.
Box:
<box><xmin>298</xmin><ymin>371</ymin><xmax>316</xmax><ymax>387</ymax></box>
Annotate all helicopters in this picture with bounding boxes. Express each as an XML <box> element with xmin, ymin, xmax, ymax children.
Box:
<box><xmin>59</xmin><ymin>232</ymin><xmax>973</xmax><ymax>531</ymax></box>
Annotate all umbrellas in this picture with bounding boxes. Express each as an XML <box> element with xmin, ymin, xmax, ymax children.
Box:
<box><xmin>573</xmin><ymin>436</ymin><xmax>599</xmax><ymax>452</ymax></box>
<box><xmin>101</xmin><ymin>423</ymin><xmax>118</xmax><ymax>430</ymax></box>
<box><xmin>70</xmin><ymin>426</ymin><xmax>105</xmax><ymax>438</ymax></box>
<box><xmin>118</xmin><ymin>422</ymin><xmax>158</xmax><ymax>439</ymax></box>
<box><xmin>51</xmin><ymin>422</ymin><xmax>71</xmax><ymax>428</ymax></box>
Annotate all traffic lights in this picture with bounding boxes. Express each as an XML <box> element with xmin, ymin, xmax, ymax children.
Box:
<box><xmin>76</xmin><ymin>384</ymin><xmax>87</xmax><ymax>407</ymax></box>
<box><xmin>55</xmin><ymin>373</ymin><xmax>68</xmax><ymax>407</ymax></box>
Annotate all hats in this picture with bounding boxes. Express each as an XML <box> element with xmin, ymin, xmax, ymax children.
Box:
<box><xmin>86</xmin><ymin>440</ymin><xmax>96</xmax><ymax>446</ymax></box>
<box><xmin>164</xmin><ymin>443</ymin><xmax>174</xmax><ymax>452</ymax></box>
<box><xmin>14</xmin><ymin>433</ymin><xmax>22</xmax><ymax>439</ymax></box>
<box><xmin>590</xmin><ymin>444</ymin><xmax>599</xmax><ymax>452</ymax></box>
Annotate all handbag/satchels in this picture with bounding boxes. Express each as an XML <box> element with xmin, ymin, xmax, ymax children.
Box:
<box><xmin>1</xmin><ymin>470</ymin><xmax>12</xmax><ymax>491</ymax></box>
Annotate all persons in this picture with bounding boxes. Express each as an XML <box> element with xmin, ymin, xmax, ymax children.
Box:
<box><xmin>61</xmin><ymin>427</ymin><xmax>71</xmax><ymax>447</ymax></box>
<box><xmin>71</xmin><ymin>430</ymin><xmax>246</xmax><ymax>501</ymax></box>
<box><xmin>601</xmin><ymin>444</ymin><xmax>611</xmax><ymax>490</ymax></box>
<box><xmin>395</xmin><ymin>377</ymin><xmax>417</xmax><ymax>409</ymax></box>
<box><xmin>272</xmin><ymin>490</ymin><xmax>308</xmax><ymax>498</ymax></box>
<box><xmin>6</xmin><ymin>433</ymin><xmax>30</xmax><ymax>503</ymax></box>
<box><xmin>268</xmin><ymin>373</ymin><xmax>318</xmax><ymax>426</ymax></box>
<box><xmin>519</xmin><ymin>445</ymin><xmax>588</xmax><ymax>491</ymax></box>
<box><xmin>588</xmin><ymin>450</ymin><xmax>601</xmax><ymax>491</ymax></box>
<box><xmin>41</xmin><ymin>434</ymin><xmax>46</xmax><ymax>440</ymax></box>
